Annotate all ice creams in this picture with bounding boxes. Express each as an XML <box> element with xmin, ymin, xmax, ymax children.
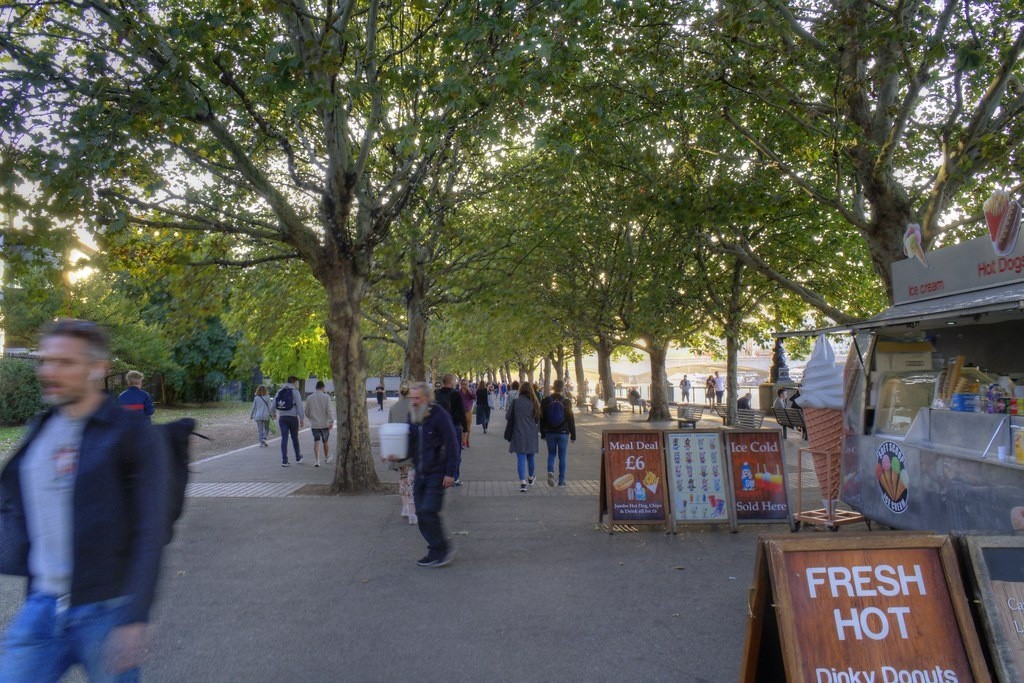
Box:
<box><xmin>903</xmin><ymin>223</ymin><xmax>929</xmax><ymax>269</ymax></box>
<box><xmin>876</xmin><ymin>455</ymin><xmax>909</xmax><ymax>501</ymax></box>
<box><xmin>794</xmin><ymin>334</ymin><xmax>844</xmax><ymax>515</ymax></box>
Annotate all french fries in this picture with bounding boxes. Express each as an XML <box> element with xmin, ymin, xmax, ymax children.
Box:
<box><xmin>983</xmin><ymin>188</ymin><xmax>1009</xmax><ymax>215</ymax></box>
<box><xmin>643</xmin><ymin>472</ymin><xmax>659</xmax><ymax>485</ymax></box>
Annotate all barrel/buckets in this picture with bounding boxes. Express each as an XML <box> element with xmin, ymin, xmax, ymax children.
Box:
<box><xmin>378</xmin><ymin>422</ymin><xmax>410</xmax><ymax>460</ymax></box>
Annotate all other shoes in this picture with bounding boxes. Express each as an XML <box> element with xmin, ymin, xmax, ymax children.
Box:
<box><xmin>548</xmin><ymin>474</ymin><xmax>554</xmax><ymax>487</ymax></box>
<box><xmin>409</xmin><ymin>515</ymin><xmax>418</xmax><ymax>525</ymax></box>
<box><xmin>325</xmin><ymin>452</ymin><xmax>333</xmax><ymax>464</ymax></box>
<box><xmin>454</xmin><ymin>480</ymin><xmax>464</xmax><ymax>485</ymax></box>
<box><xmin>314</xmin><ymin>461</ymin><xmax>320</xmax><ymax>467</ymax></box>
<box><xmin>401</xmin><ymin>507</ymin><xmax>409</xmax><ymax>517</ymax></box>
<box><xmin>296</xmin><ymin>455</ymin><xmax>304</xmax><ymax>464</ymax></box>
<box><xmin>462</xmin><ymin>441</ymin><xmax>469</xmax><ymax>449</ymax></box>
<box><xmin>417</xmin><ymin>548</ymin><xmax>456</xmax><ymax>567</ymax></box>
<box><xmin>520</xmin><ymin>484</ymin><xmax>527</xmax><ymax>492</ymax></box>
<box><xmin>260</xmin><ymin>439</ymin><xmax>268</xmax><ymax>446</ymax></box>
<box><xmin>281</xmin><ymin>462</ymin><xmax>291</xmax><ymax>467</ymax></box>
<box><xmin>558</xmin><ymin>482</ymin><xmax>567</xmax><ymax>488</ymax></box>
<box><xmin>528</xmin><ymin>475</ymin><xmax>536</xmax><ymax>486</ymax></box>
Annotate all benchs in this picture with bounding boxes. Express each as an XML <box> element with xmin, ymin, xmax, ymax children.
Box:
<box><xmin>713</xmin><ymin>402</ymin><xmax>809</xmax><ymax>441</ymax></box>
<box><xmin>626</xmin><ymin>396</ymin><xmax>646</xmax><ymax>414</ymax></box>
<box><xmin>601</xmin><ymin>398</ymin><xmax>617</xmax><ymax>415</ymax></box>
<box><xmin>582</xmin><ymin>396</ymin><xmax>596</xmax><ymax>413</ymax></box>
<box><xmin>669</xmin><ymin>405</ymin><xmax>704</xmax><ymax>429</ymax></box>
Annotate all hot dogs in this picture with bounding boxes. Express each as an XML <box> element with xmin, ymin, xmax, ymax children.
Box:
<box><xmin>995</xmin><ymin>201</ymin><xmax>1022</xmax><ymax>253</ymax></box>
<box><xmin>613</xmin><ymin>473</ymin><xmax>634</xmax><ymax>491</ymax></box>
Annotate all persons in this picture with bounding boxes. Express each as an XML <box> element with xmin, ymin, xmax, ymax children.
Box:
<box><xmin>540</xmin><ymin>380</ymin><xmax>576</xmax><ymax>488</ymax></box>
<box><xmin>738</xmin><ymin>393</ymin><xmax>752</xmax><ymax>410</ymax></box>
<box><xmin>585</xmin><ymin>378</ymin><xmax>589</xmax><ymax>393</ymax></box>
<box><xmin>305</xmin><ymin>381</ymin><xmax>333</xmax><ymax>467</ymax></box>
<box><xmin>485</xmin><ymin>380</ymin><xmax>520</xmax><ymax>411</ymax></box>
<box><xmin>270</xmin><ymin>376</ymin><xmax>305</xmax><ymax>466</ymax></box>
<box><xmin>564</xmin><ymin>383</ymin><xmax>577</xmax><ymax>403</ymax></box>
<box><xmin>679</xmin><ymin>375</ymin><xmax>690</xmax><ymax>404</ymax></box>
<box><xmin>250</xmin><ymin>385</ymin><xmax>274</xmax><ymax>447</ymax></box>
<box><xmin>773</xmin><ymin>388</ymin><xmax>802</xmax><ymax>432</ymax></box>
<box><xmin>506</xmin><ymin>382</ymin><xmax>540</xmax><ymax>492</ymax></box>
<box><xmin>427</xmin><ymin>381</ymin><xmax>443</xmax><ymax>390</ymax></box>
<box><xmin>376</xmin><ymin>383</ymin><xmax>385</xmax><ymax>411</ymax></box>
<box><xmin>380</xmin><ymin>382</ymin><xmax>461</xmax><ymax>567</ymax></box>
<box><xmin>627</xmin><ymin>386</ymin><xmax>651</xmax><ymax>414</ymax></box>
<box><xmin>0</xmin><ymin>318</ymin><xmax>169</xmax><ymax>683</ymax></box>
<box><xmin>119</xmin><ymin>369</ymin><xmax>156</xmax><ymax>423</ymax></box>
<box><xmin>456</xmin><ymin>377</ymin><xmax>479</xmax><ymax>450</ymax></box>
<box><xmin>434</xmin><ymin>374</ymin><xmax>468</xmax><ymax>487</ymax></box>
<box><xmin>530</xmin><ymin>381</ymin><xmax>544</xmax><ymax>437</ymax></box>
<box><xmin>476</xmin><ymin>382</ymin><xmax>491</xmax><ymax>435</ymax></box>
<box><xmin>706</xmin><ymin>371</ymin><xmax>726</xmax><ymax>413</ymax></box>
<box><xmin>789</xmin><ymin>384</ymin><xmax>808</xmax><ymax>440</ymax></box>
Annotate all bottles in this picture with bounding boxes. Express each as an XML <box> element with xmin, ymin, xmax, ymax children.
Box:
<box><xmin>635</xmin><ymin>480</ymin><xmax>642</xmax><ymax>500</ymax></box>
<box><xmin>742</xmin><ymin>462</ymin><xmax>752</xmax><ymax>490</ymax></box>
<box><xmin>974</xmin><ymin>369</ymin><xmax>1024</xmax><ymax>416</ymax></box>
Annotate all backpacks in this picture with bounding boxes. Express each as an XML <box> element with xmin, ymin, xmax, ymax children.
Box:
<box><xmin>545</xmin><ymin>396</ymin><xmax>569</xmax><ymax>428</ymax></box>
<box><xmin>116</xmin><ymin>417</ymin><xmax>213</xmax><ymax>558</ymax></box>
<box><xmin>276</xmin><ymin>386</ymin><xmax>296</xmax><ymax>410</ymax></box>
<box><xmin>437</xmin><ymin>389</ymin><xmax>457</xmax><ymax>413</ymax></box>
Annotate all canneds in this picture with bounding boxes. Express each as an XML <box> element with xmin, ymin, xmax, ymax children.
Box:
<box><xmin>628</xmin><ymin>488</ymin><xmax>634</xmax><ymax>500</ymax></box>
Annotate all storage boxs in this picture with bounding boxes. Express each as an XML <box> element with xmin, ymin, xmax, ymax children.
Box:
<box><xmin>379</xmin><ymin>422</ymin><xmax>409</xmax><ymax>460</ymax></box>
<box><xmin>873</xmin><ymin>341</ymin><xmax>936</xmax><ymax>373</ymax></box>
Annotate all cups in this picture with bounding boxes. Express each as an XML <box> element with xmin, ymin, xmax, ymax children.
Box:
<box><xmin>998</xmin><ymin>447</ymin><xmax>1007</xmax><ymax>461</ymax></box>
<box><xmin>755</xmin><ymin>473</ymin><xmax>782</xmax><ymax>492</ymax></box>
<box><xmin>708</xmin><ymin>495</ymin><xmax>726</xmax><ymax>513</ymax></box>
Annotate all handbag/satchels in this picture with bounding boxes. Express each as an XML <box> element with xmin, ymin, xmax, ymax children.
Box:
<box><xmin>268</xmin><ymin>416</ymin><xmax>277</xmax><ymax>435</ymax></box>
<box><xmin>433</xmin><ymin>462</ymin><xmax>459</xmax><ymax>487</ymax></box>
<box><xmin>504</xmin><ymin>399</ymin><xmax>516</xmax><ymax>442</ymax></box>
<box><xmin>487</xmin><ymin>388</ymin><xmax>494</xmax><ymax>408</ymax></box>
